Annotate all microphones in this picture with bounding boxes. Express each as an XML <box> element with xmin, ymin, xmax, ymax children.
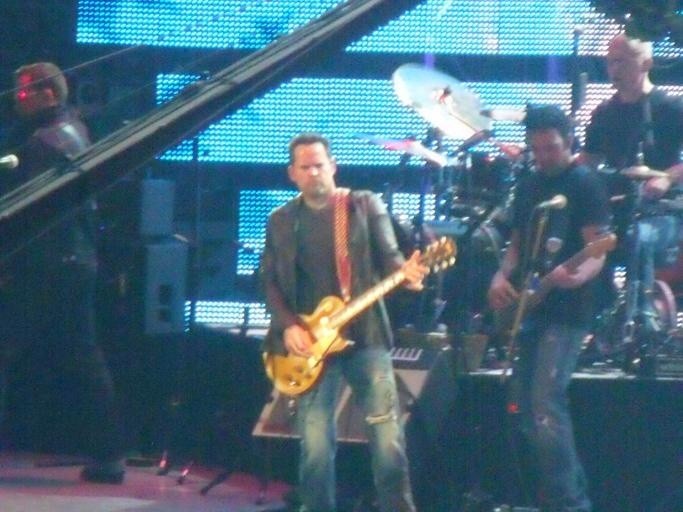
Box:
<box><xmin>570</xmin><ymin>71</ymin><xmax>587</xmax><ymax>113</ymax></box>
<box><xmin>456</xmin><ymin>129</ymin><xmax>490</xmax><ymax>153</ymax></box>
<box><xmin>0</xmin><ymin>154</ymin><xmax>20</xmax><ymax>169</ymax></box>
<box><xmin>535</xmin><ymin>194</ymin><xmax>567</xmax><ymax>210</ymax></box>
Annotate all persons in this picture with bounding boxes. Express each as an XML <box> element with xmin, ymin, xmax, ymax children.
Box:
<box><xmin>485</xmin><ymin>103</ymin><xmax>617</xmax><ymax>512</ymax></box>
<box><xmin>262</xmin><ymin>135</ymin><xmax>431</xmax><ymax>512</ymax></box>
<box><xmin>501</xmin><ymin>32</ymin><xmax>683</xmax><ymax>339</ymax></box>
<box><xmin>2</xmin><ymin>62</ymin><xmax>128</xmax><ymax>486</ymax></box>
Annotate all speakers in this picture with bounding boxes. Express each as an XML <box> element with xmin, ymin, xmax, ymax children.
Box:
<box><xmin>96</xmin><ymin>180</ymin><xmax>189</xmax><ymax>342</ymax></box>
<box><xmin>251</xmin><ymin>347</ymin><xmax>461</xmax><ymax>471</ymax></box>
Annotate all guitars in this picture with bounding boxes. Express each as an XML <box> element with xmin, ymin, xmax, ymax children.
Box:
<box><xmin>262</xmin><ymin>236</ymin><xmax>457</xmax><ymax>397</ymax></box>
<box><xmin>469</xmin><ymin>235</ymin><xmax>618</xmax><ymax>350</ymax></box>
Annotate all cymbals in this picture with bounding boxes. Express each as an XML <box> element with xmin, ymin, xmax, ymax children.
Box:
<box><xmin>596</xmin><ymin>168</ymin><xmax>682</xmax><ymax>179</ymax></box>
<box><xmin>354</xmin><ymin>134</ymin><xmax>451</xmax><ymax>166</ymax></box>
<box><xmin>485</xmin><ymin>107</ymin><xmax>526</xmax><ymax>123</ymax></box>
<box><xmin>392</xmin><ymin>63</ymin><xmax>494</xmax><ymax>139</ymax></box>
